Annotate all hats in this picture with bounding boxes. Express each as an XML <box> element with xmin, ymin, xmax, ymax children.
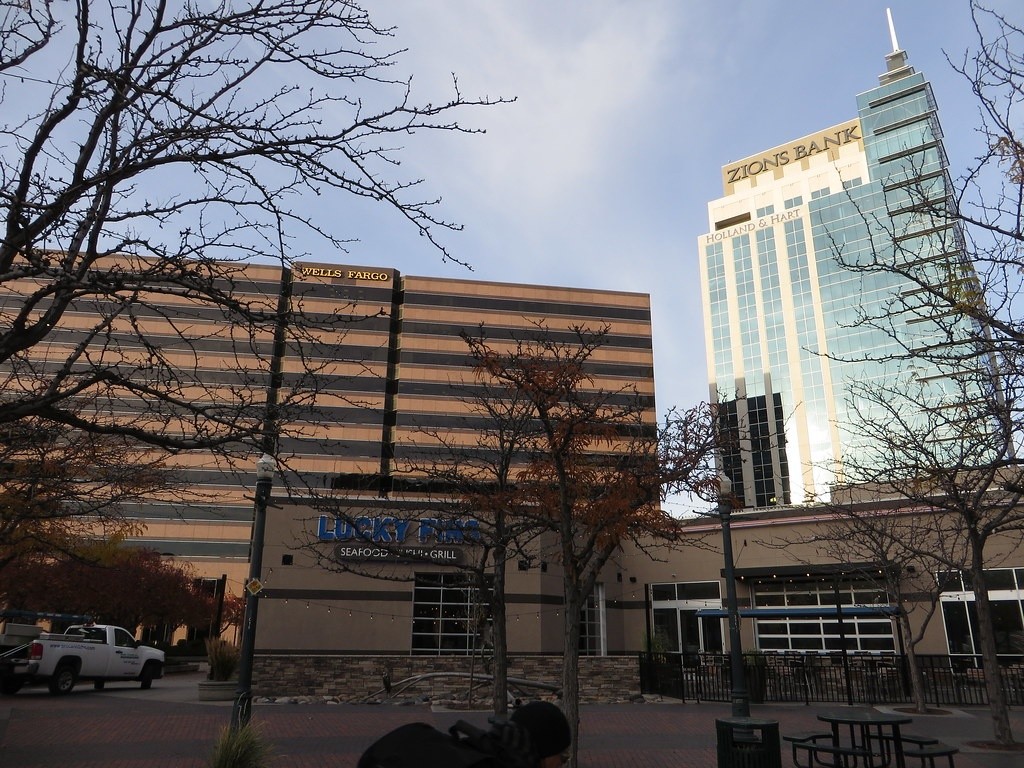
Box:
<box><xmin>510</xmin><ymin>701</ymin><xmax>571</xmax><ymax>758</ymax></box>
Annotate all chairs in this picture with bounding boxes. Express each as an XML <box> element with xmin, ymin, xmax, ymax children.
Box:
<box><xmin>683</xmin><ymin>651</ymin><xmax>965</xmax><ymax>703</ymax></box>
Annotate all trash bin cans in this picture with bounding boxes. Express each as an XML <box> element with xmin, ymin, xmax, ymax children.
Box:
<box><xmin>714</xmin><ymin>716</ymin><xmax>783</xmax><ymax>768</ymax></box>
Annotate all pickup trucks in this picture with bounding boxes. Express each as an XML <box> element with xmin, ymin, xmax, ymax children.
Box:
<box><xmin>5</xmin><ymin>624</ymin><xmax>168</xmax><ymax>693</ymax></box>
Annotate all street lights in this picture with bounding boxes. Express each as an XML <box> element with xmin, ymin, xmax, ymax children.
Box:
<box><xmin>712</xmin><ymin>469</ymin><xmax>762</xmax><ymax>744</ymax></box>
<box><xmin>230</xmin><ymin>452</ymin><xmax>278</xmax><ymax>735</ymax></box>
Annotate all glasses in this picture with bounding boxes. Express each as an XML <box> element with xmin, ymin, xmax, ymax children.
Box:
<box><xmin>558</xmin><ymin>751</ymin><xmax>571</xmax><ymax>765</ymax></box>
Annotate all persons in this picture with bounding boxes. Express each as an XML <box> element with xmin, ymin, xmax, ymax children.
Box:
<box><xmin>456</xmin><ymin>700</ymin><xmax>573</xmax><ymax>768</ymax></box>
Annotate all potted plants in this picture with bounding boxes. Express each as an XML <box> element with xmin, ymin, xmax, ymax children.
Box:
<box><xmin>197</xmin><ymin>635</ymin><xmax>239</xmax><ymax>700</ymax></box>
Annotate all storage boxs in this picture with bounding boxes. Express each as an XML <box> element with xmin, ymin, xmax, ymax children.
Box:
<box><xmin>0</xmin><ymin>623</ymin><xmax>103</xmax><ymax>658</ymax></box>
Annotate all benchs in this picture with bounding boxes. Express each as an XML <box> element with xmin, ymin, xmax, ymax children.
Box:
<box><xmin>783</xmin><ymin>730</ymin><xmax>959</xmax><ymax>768</ymax></box>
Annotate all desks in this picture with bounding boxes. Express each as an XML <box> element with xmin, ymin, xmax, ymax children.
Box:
<box><xmin>817</xmin><ymin>712</ymin><xmax>912</xmax><ymax>768</ymax></box>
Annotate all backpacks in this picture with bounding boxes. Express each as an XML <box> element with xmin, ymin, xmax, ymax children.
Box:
<box><xmin>357</xmin><ymin>721</ymin><xmax>507</xmax><ymax>768</ymax></box>
